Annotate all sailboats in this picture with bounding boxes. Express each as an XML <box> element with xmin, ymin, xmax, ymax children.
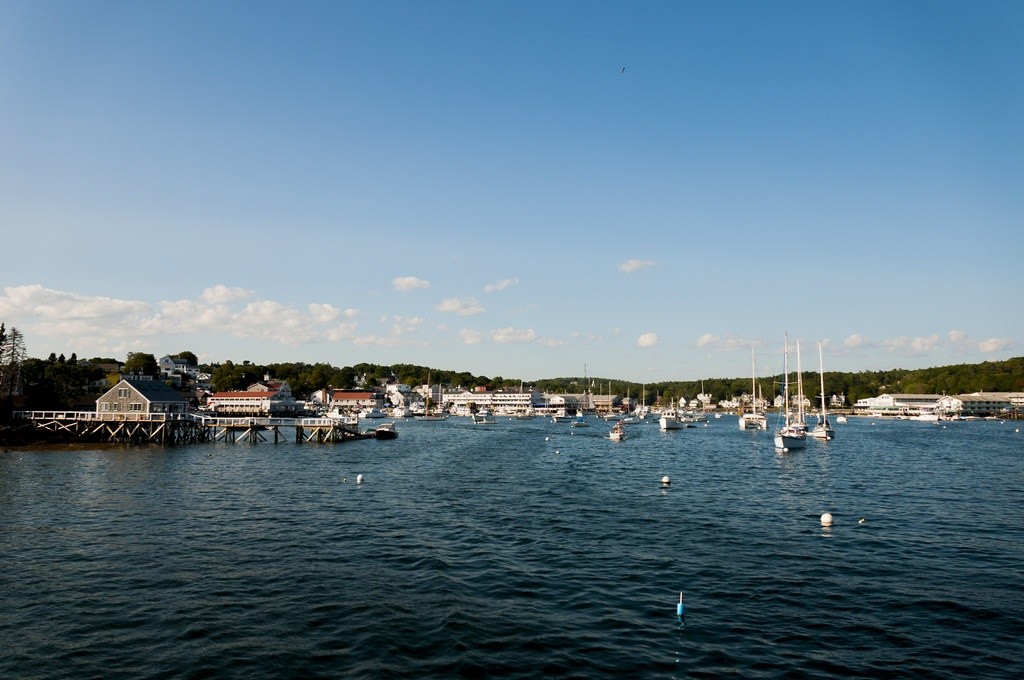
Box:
<box><xmin>593</xmin><ymin>380</ymin><xmax>723</xmax><ymax>430</ymax></box>
<box><xmin>739</xmin><ymin>330</ymin><xmax>836</xmax><ymax>450</ymax></box>
<box><xmin>353</xmin><ymin>367</ymin><xmax>457</xmax><ymax>424</ymax></box>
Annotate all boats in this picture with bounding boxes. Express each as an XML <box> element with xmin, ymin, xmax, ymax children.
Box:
<box><xmin>515</xmin><ymin>406</ymin><xmax>535</xmax><ymax>421</ymax></box>
<box><xmin>608</xmin><ymin>420</ymin><xmax>627</xmax><ymax>441</ymax></box>
<box><xmin>470</xmin><ymin>399</ymin><xmax>498</xmax><ymax>424</ymax></box>
<box><xmin>571</xmin><ymin>416</ymin><xmax>590</xmax><ymax>428</ymax></box>
<box><xmin>552</xmin><ymin>405</ymin><xmax>572</xmax><ymax>424</ymax></box>
